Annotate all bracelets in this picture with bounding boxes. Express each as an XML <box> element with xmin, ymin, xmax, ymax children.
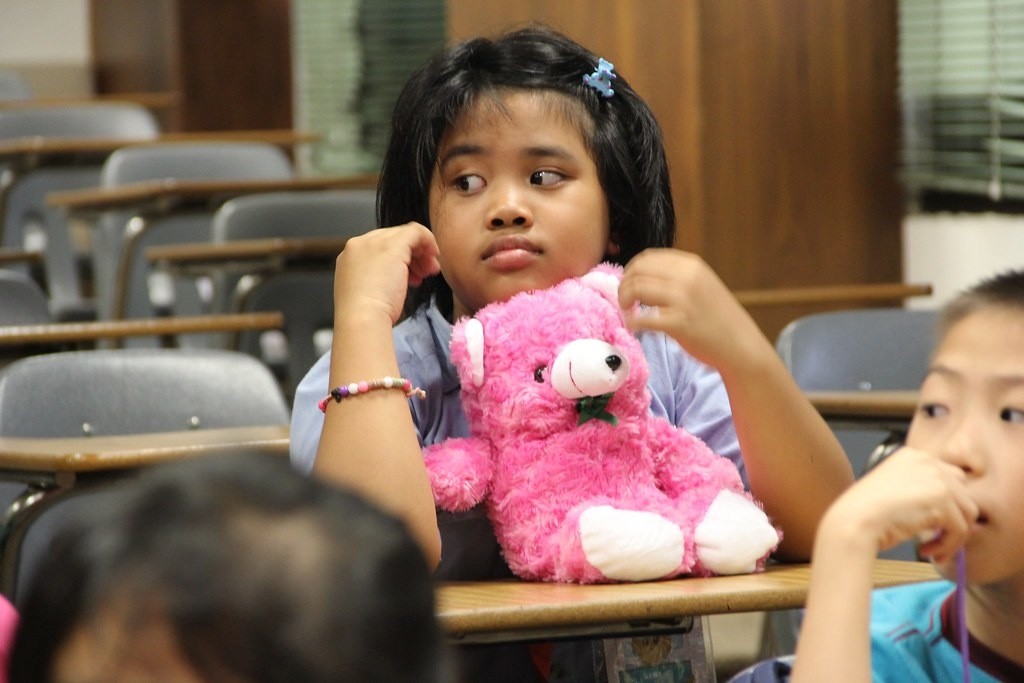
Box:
<box><xmin>317</xmin><ymin>377</ymin><xmax>427</xmax><ymax>410</ymax></box>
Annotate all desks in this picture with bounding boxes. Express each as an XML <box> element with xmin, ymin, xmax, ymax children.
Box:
<box><xmin>736</xmin><ymin>285</ymin><xmax>931</xmax><ymax>306</ymax></box>
<box><xmin>47</xmin><ymin>176</ymin><xmax>381</xmax><ymax>318</ymax></box>
<box><xmin>1</xmin><ymin>425</ymin><xmax>293</xmax><ymax>605</ymax></box>
<box><xmin>430</xmin><ymin>560</ymin><xmax>941</xmax><ymax>646</ymax></box>
<box><xmin>0</xmin><ymin>310</ymin><xmax>285</xmax><ymax>343</ymax></box>
<box><xmin>1</xmin><ymin>133</ymin><xmax>321</xmax><ymax>176</ymax></box>
<box><xmin>807</xmin><ymin>392</ymin><xmax>922</xmax><ymax>431</ymax></box>
<box><xmin>146</xmin><ymin>235</ymin><xmax>366</xmax><ymax>351</ymax></box>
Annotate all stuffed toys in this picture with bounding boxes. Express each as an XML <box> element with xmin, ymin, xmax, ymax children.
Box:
<box><xmin>422</xmin><ymin>261</ymin><xmax>790</xmax><ymax>583</ymax></box>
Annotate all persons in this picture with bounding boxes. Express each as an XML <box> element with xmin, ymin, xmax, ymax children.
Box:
<box><xmin>286</xmin><ymin>20</ymin><xmax>859</xmax><ymax>585</ymax></box>
<box><xmin>726</xmin><ymin>271</ymin><xmax>1023</xmax><ymax>683</ymax></box>
<box><xmin>9</xmin><ymin>453</ymin><xmax>437</xmax><ymax>683</ymax></box>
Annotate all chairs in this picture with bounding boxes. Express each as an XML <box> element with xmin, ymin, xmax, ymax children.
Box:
<box><xmin>1</xmin><ymin>62</ymin><xmax>1024</xmax><ymax>683</ymax></box>
